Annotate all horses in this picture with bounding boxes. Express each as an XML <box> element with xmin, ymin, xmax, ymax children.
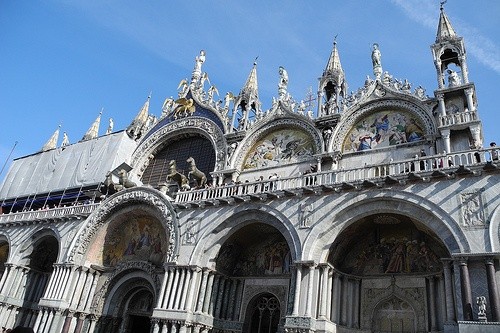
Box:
<box><xmin>165</xmin><ymin>159</ymin><xmax>190</xmax><ymax>192</ymax></box>
<box><xmin>99</xmin><ymin>170</ymin><xmax>120</xmax><ymax>201</ymax></box>
<box><xmin>112</xmin><ymin>169</ymin><xmax>137</xmax><ymax>192</ymax></box>
<box><xmin>186</xmin><ymin>157</ymin><xmax>208</xmax><ymax>190</ymax></box>
